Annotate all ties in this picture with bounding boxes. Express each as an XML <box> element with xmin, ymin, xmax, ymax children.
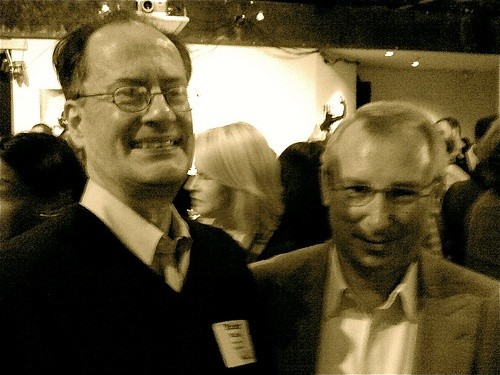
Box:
<box><xmin>158</xmin><ymin>241</ymin><xmax>185</xmax><ymax>294</ymax></box>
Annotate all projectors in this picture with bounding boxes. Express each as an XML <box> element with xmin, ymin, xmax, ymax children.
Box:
<box><xmin>135</xmin><ymin>0</ymin><xmax>189</xmax><ymax>37</ymax></box>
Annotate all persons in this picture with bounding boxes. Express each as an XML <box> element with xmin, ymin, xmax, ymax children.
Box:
<box><xmin>247</xmin><ymin>100</ymin><xmax>500</xmax><ymax>375</ymax></box>
<box><xmin>0</xmin><ymin>114</ymin><xmax>500</xmax><ymax>281</ymax></box>
<box><xmin>0</xmin><ymin>8</ymin><xmax>278</xmax><ymax>375</ymax></box>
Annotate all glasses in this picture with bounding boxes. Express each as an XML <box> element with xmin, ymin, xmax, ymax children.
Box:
<box><xmin>330</xmin><ymin>179</ymin><xmax>441</xmax><ymax>207</ymax></box>
<box><xmin>76</xmin><ymin>84</ymin><xmax>200</xmax><ymax>113</ymax></box>
